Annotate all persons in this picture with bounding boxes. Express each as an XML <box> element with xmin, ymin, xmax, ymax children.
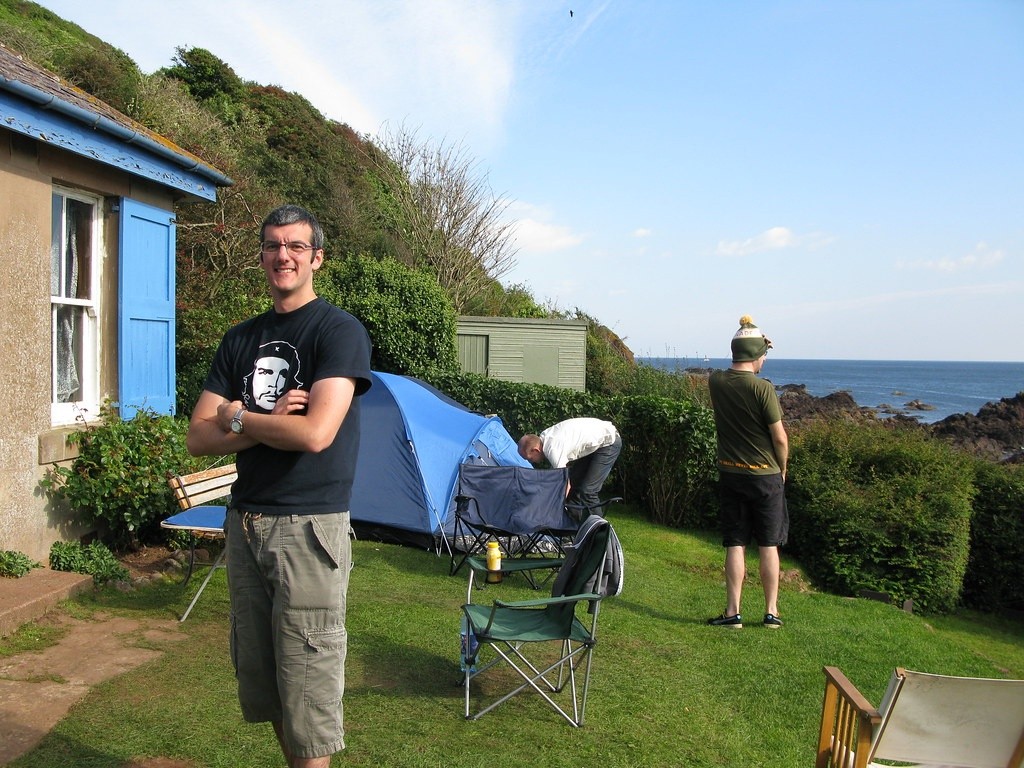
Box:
<box><xmin>187</xmin><ymin>205</ymin><xmax>374</xmax><ymax>768</ymax></box>
<box><xmin>709</xmin><ymin>316</ymin><xmax>788</xmax><ymax>628</ymax></box>
<box><xmin>519</xmin><ymin>418</ymin><xmax>622</xmax><ymax>547</ymax></box>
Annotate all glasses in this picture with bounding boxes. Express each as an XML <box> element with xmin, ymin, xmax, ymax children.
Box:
<box><xmin>260</xmin><ymin>241</ymin><xmax>321</xmax><ymax>256</ymax></box>
<box><xmin>764</xmin><ymin>350</ymin><xmax>768</xmax><ymax>356</ymax></box>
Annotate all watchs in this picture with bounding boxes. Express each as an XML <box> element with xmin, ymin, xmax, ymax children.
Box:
<box><xmin>230</xmin><ymin>409</ymin><xmax>248</xmax><ymax>434</ymax></box>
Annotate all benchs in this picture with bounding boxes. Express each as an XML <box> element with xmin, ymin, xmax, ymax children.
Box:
<box><xmin>449</xmin><ymin>462</ymin><xmax>624</xmax><ymax>590</ymax></box>
<box><xmin>168</xmin><ymin>463</ymin><xmax>238</xmax><ymax>588</ymax></box>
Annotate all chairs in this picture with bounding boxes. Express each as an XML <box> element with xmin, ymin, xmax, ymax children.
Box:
<box><xmin>815</xmin><ymin>665</ymin><xmax>1024</xmax><ymax>768</ymax></box>
<box><xmin>460</xmin><ymin>514</ymin><xmax>624</xmax><ymax>729</ymax></box>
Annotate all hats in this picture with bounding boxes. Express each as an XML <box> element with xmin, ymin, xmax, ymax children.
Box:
<box><xmin>731</xmin><ymin>315</ymin><xmax>768</xmax><ymax>362</ymax></box>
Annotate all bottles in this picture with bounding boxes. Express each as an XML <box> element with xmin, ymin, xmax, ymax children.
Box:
<box><xmin>487</xmin><ymin>541</ymin><xmax>502</xmax><ymax>584</ymax></box>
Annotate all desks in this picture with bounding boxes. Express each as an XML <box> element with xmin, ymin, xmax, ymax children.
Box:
<box><xmin>161</xmin><ymin>505</ymin><xmax>226</xmax><ymax>623</ymax></box>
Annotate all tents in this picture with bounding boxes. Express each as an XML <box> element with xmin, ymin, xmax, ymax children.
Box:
<box><xmin>350</xmin><ymin>371</ymin><xmax>565</xmax><ymax>555</ymax></box>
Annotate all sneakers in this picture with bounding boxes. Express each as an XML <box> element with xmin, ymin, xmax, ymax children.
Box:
<box><xmin>763</xmin><ymin>613</ymin><xmax>782</xmax><ymax>628</ymax></box>
<box><xmin>708</xmin><ymin>608</ymin><xmax>742</xmax><ymax>629</ymax></box>
